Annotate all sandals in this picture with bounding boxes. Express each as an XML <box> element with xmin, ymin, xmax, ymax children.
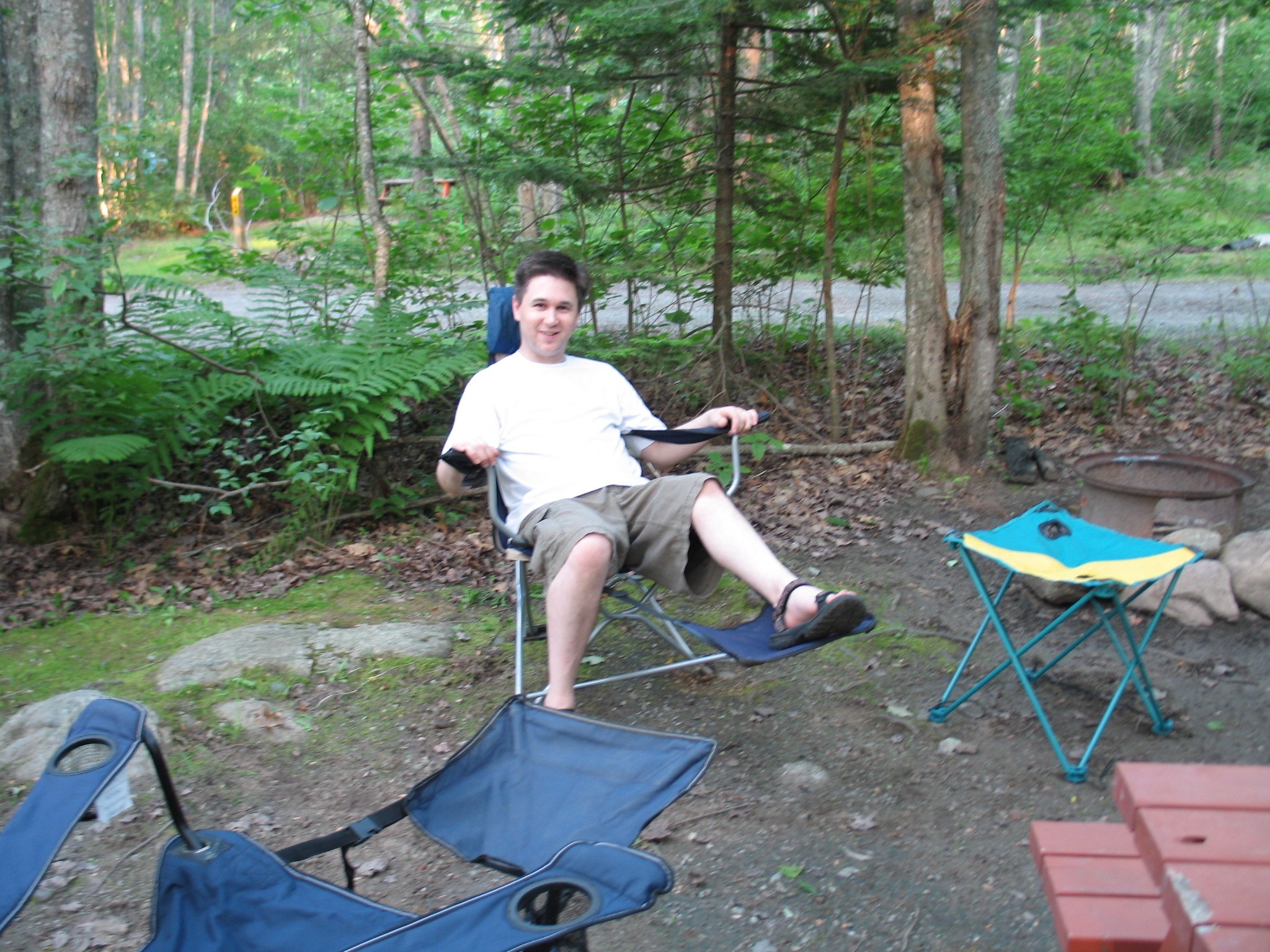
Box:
<box><xmin>770</xmin><ymin>577</ymin><xmax>867</xmax><ymax>650</ymax></box>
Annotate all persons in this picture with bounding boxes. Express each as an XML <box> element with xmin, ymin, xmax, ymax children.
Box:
<box><xmin>435</xmin><ymin>250</ymin><xmax>864</xmax><ymax>712</ymax></box>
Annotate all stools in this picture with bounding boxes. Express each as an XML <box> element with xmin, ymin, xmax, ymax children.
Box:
<box><xmin>924</xmin><ymin>499</ymin><xmax>1205</xmax><ymax>785</ymax></box>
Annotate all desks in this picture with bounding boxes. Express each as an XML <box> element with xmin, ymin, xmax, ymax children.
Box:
<box><xmin>1112</xmin><ymin>758</ymin><xmax>1270</xmax><ymax>952</ymax></box>
<box><xmin>378</xmin><ymin>177</ymin><xmax>463</xmax><ymax>213</ymax></box>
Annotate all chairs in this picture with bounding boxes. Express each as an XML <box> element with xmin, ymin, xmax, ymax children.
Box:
<box><xmin>439</xmin><ymin>285</ymin><xmax>881</xmax><ymax>706</ymax></box>
<box><xmin>0</xmin><ymin>692</ymin><xmax>716</xmax><ymax>952</ymax></box>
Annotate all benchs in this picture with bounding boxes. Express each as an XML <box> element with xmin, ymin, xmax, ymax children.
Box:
<box><xmin>378</xmin><ymin>197</ymin><xmax>408</xmax><ymax>206</ymax></box>
<box><xmin>1028</xmin><ymin>819</ymin><xmax>1171</xmax><ymax>952</ymax></box>
